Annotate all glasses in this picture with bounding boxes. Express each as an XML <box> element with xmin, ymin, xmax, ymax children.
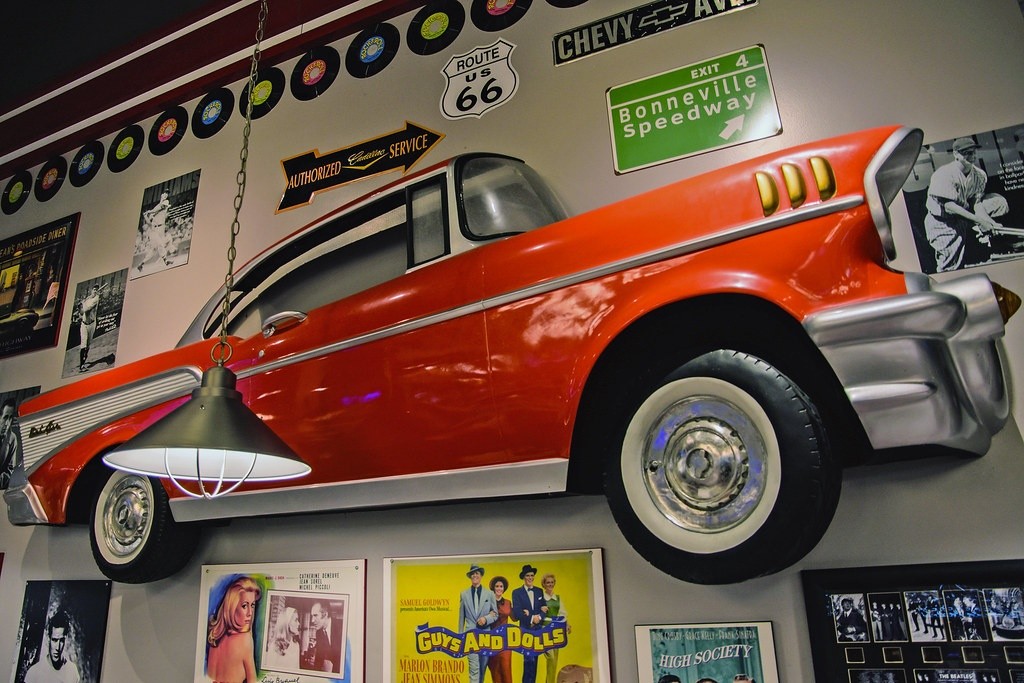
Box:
<box><xmin>959</xmin><ymin>151</ymin><xmax>976</xmax><ymax>155</ymax></box>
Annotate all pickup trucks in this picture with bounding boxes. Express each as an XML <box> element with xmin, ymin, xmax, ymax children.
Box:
<box><xmin>1</xmin><ymin>126</ymin><xmax>1024</xmax><ymax>585</ymax></box>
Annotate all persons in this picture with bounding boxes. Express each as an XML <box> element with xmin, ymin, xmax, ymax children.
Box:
<box><xmin>833</xmin><ymin>595</ymin><xmax>1022</xmax><ymax>642</ymax></box>
<box><xmin>24</xmin><ymin>615</ymin><xmax>80</xmax><ymax>683</ymax></box>
<box><xmin>135</xmin><ymin>188</ymin><xmax>174</xmax><ymax>272</ymax></box>
<box><xmin>77</xmin><ymin>283</ymin><xmax>99</xmax><ymax>372</ymax></box>
<box><xmin>311</xmin><ymin>599</ymin><xmax>344</xmax><ymax>674</ymax></box>
<box><xmin>205</xmin><ymin>577</ymin><xmax>262</xmax><ymax>683</ymax></box>
<box><xmin>925</xmin><ymin>138</ymin><xmax>1003</xmax><ymax>272</ymax></box>
<box><xmin>0</xmin><ymin>397</ymin><xmax>18</xmax><ymax>490</ymax></box>
<box><xmin>458</xmin><ymin>563</ymin><xmax>572</xmax><ymax>683</ymax></box>
<box><xmin>267</xmin><ymin>607</ymin><xmax>300</xmax><ymax>669</ymax></box>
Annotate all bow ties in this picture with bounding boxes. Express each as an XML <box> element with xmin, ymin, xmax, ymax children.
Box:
<box><xmin>528</xmin><ymin>588</ymin><xmax>534</xmax><ymax>591</ymax></box>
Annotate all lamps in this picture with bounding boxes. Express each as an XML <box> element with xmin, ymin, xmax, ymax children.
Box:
<box><xmin>102</xmin><ymin>0</ymin><xmax>313</xmax><ymax>499</ymax></box>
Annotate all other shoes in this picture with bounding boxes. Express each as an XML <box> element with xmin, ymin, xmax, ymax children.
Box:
<box><xmin>923</xmin><ymin>631</ymin><xmax>928</xmax><ymax>634</ymax></box>
<box><xmin>166</xmin><ymin>260</ymin><xmax>174</xmax><ymax>265</ymax></box>
<box><xmin>1005</xmin><ymin>243</ymin><xmax>1023</xmax><ymax>252</ymax></box>
<box><xmin>916</xmin><ymin>629</ymin><xmax>920</xmax><ymax>631</ymax></box>
<box><xmin>932</xmin><ymin>636</ymin><xmax>937</xmax><ymax>638</ymax></box>
<box><xmin>138</xmin><ymin>262</ymin><xmax>144</xmax><ymax>272</ymax></box>
<box><xmin>942</xmin><ymin>638</ymin><xmax>945</xmax><ymax>640</ymax></box>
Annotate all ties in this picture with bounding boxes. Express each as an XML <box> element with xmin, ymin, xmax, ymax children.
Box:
<box><xmin>846</xmin><ymin>614</ymin><xmax>847</xmax><ymax>618</ymax></box>
<box><xmin>325</xmin><ymin>631</ymin><xmax>329</xmax><ymax>644</ymax></box>
<box><xmin>474</xmin><ymin>588</ymin><xmax>478</xmax><ymax>614</ymax></box>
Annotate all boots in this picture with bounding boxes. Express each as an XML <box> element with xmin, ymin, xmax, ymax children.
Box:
<box><xmin>82</xmin><ymin>348</ymin><xmax>89</xmax><ymax>369</ymax></box>
<box><xmin>79</xmin><ymin>348</ymin><xmax>86</xmax><ymax>373</ymax></box>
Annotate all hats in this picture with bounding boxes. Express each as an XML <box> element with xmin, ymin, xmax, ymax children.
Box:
<box><xmin>162</xmin><ymin>188</ymin><xmax>170</xmax><ymax>194</ymax></box>
<box><xmin>489</xmin><ymin>576</ymin><xmax>508</xmax><ymax>591</ymax></box>
<box><xmin>519</xmin><ymin>565</ymin><xmax>537</xmax><ymax>579</ymax></box>
<box><xmin>92</xmin><ymin>283</ymin><xmax>99</xmax><ymax>290</ymax></box>
<box><xmin>953</xmin><ymin>138</ymin><xmax>983</xmax><ymax>150</ymax></box>
<box><xmin>466</xmin><ymin>565</ymin><xmax>484</xmax><ymax>578</ymax></box>
